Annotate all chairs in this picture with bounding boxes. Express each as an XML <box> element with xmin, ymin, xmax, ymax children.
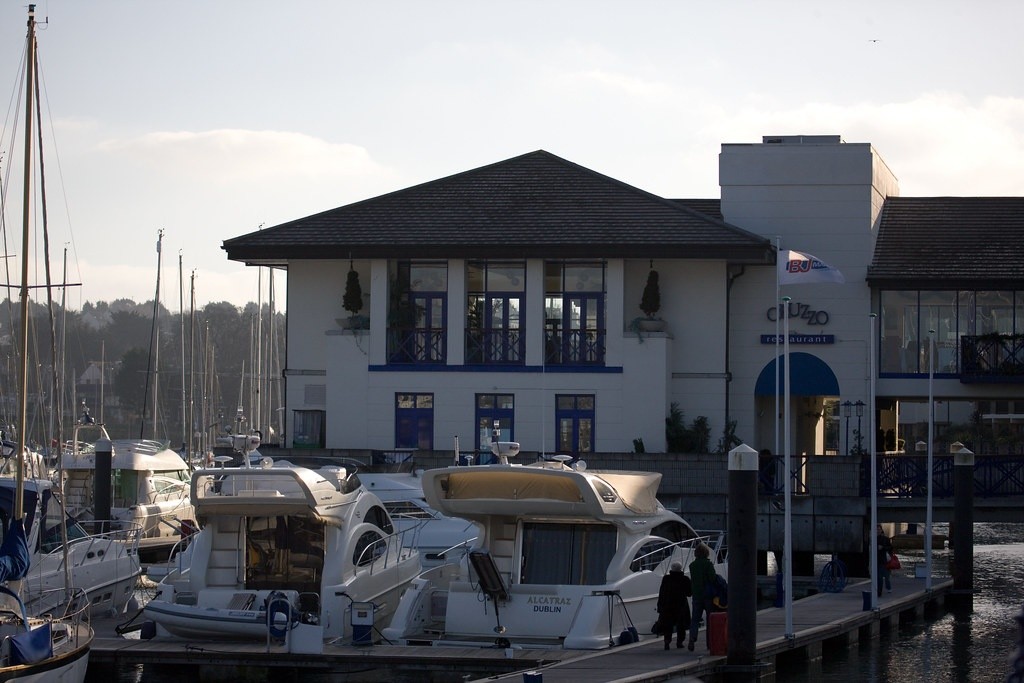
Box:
<box><xmin>899</xmin><ymin>341</ymin><xmax>920</xmax><ymax>372</ymax></box>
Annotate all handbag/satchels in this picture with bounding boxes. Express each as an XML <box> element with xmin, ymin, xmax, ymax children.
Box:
<box><xmin>885</xmin><ymin>552</ymin><xmax>901</xmax><ymax>569</ymax></box>
<box><xmin>652</xmin><ymin>617</ymin><xmax>665</xmax><ymax>636</ymax></box>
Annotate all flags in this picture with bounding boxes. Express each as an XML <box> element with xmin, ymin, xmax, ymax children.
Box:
<box><xmin>777</xmin><ymin>250</ymin><xmax>845</xmax><ymax>285</ymax></box>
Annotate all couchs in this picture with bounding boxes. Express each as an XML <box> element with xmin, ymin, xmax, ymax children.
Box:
<box><xmin>925</xmin><ymin>341</ymin><xmax>960</xmax><ymax>373</ymax></box>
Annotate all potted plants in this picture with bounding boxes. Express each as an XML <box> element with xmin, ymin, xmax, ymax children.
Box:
<box><xmin>333</xmin><ymin>260</ymin><xmax>366</xmax><ymax>330</ymax></box>
<box><xmin>628</xmin><ymin>270</ymin><xmax>668</xmax><ymax>348</ymax></box>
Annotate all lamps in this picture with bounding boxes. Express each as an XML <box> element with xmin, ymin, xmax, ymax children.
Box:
<box><xmin>813</xmin><ymin>412</ymin><xmax>822</xmax><ymax>418</ymax></box>
<box><xmin>758</xmin><ymin>410</ymin><xmax>766</xmax><ymax>417</ymax></box>
<box><xmin>572</xmin><ymin>461</ymin><xmax>587</xmax><ymax>472</ymax></box>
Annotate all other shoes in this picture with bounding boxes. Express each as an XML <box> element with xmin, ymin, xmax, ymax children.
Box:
<box><xmin>887</xmin><ymin>589</ymin><xmax>892</xmax><ymax>593</ymax></box>
<box><xmin>688</xmin><ymin>642</ymin><xmax>694</xmax><ymax>651</ymax></box>
<box><xmin>677</xmin><ymin>643</ymin><xmax>685</xmax><ymax>648</ymax></box>
<box><xmin>665</xmin><ymin>644</ymin><xmax>670</xmax><ymax>650</ymax></box>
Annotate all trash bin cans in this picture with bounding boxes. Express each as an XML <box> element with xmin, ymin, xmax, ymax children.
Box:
<box><xmin>861</xmin><ymin>590</ymin><xmax>872</xmax><ymax>612</ymax></box>
<box><xmin>708</xmin><ymin>611</ymin><xmax>727</xmax><ymax>656</ymax></box>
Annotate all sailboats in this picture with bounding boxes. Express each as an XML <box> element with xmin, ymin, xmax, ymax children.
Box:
<box><xmin>1</xmin><ymin>0</ymin><xmax>784</xmax><ymax>683</ymax></box>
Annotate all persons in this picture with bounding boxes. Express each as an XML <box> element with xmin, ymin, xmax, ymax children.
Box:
<box><xmin>657</xmin><ymin>561</ymin><xmax>692</xmax><ymax>650</ymax></box>
<box><xmin>862</xmin><ymin>525</ymin><xmax>893</xmax><ymax>597</ymax></box>
<box><xmin>687</xmin><ymin>544</ymin><xmax>717</xmax><ymax>651</ymax></box>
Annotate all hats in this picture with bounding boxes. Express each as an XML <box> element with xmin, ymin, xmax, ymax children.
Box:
<box><xmin>671</xmin><ymin>562</ymin><xmax>683</xmax><ymax>571</ymax></box>
